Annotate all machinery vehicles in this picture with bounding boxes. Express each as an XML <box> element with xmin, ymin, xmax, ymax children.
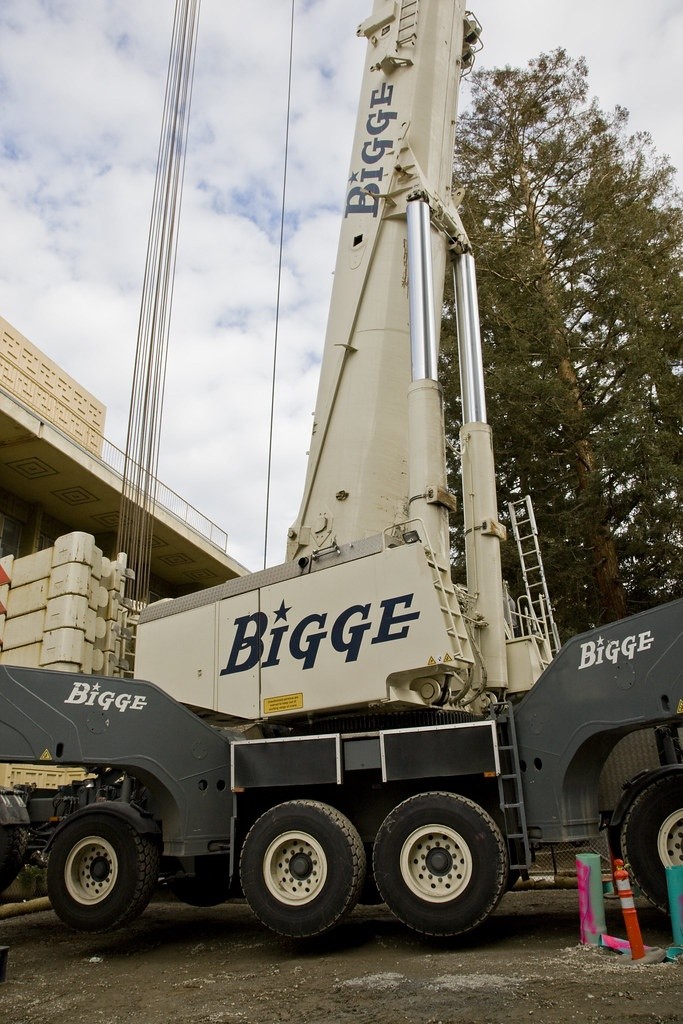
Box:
<box><xmin>0</xmin><ymin>0</ymin><xmax>683</xmax><ymax>953</ymax></box>
